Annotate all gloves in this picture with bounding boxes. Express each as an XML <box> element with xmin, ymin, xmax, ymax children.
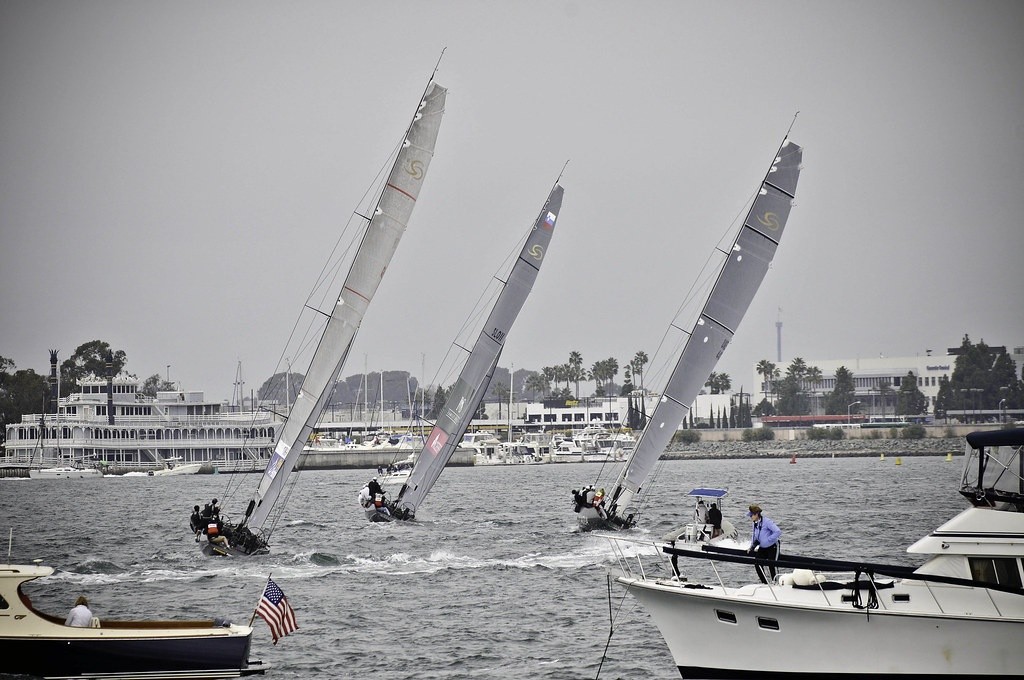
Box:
<box><xmin>746</xmin><ymin>546</ymin><xmax>756</xmax><ymax>556</ymax></box>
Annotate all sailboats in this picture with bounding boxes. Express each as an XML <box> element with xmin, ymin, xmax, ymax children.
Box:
<box><xmin>364</xmin><ymin>157</ymin><xmax>572</xmax><ymax>524</ymax></box>
<box><xmin>573</xmin><ymin>108</ymin><xmax>813</xmax><ymax>532</ymax></box>
<box><xmin>201</xmin><ymin>44</ymin><xmax>457</xmax><ymax>560</ymax></box>
<box><xmin>288</xmin><ymin>356</ymin><xmax>643</xmax><ymax>465</ymax></box>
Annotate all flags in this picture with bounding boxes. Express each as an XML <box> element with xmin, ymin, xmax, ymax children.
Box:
<box><xmin>255</xmin><ymin>577</ymin><xmax>301</xmax><ymax>646</ymax></box>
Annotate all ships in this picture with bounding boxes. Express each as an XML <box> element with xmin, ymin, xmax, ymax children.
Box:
<box><xmin>0</xmin><ymin>366</ymin><xmax>295</xmax><ymax>473</ymax></box>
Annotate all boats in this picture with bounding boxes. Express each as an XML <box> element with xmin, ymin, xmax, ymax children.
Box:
<box><xmin>592</xmin><ymin>428</ymin><xmax>1023</xmax><ymax>680</ymax></box>
<box><xmin>0</xmin><ymin>561</ymin><xmax>271</xmax><ymax>680</ymax></box>
<box><xmin>661</xmin><ymin>485</ymin><xmax>743</xmax><ymax>554</ymax></box>
<box><xmin>144</xmin><ymin>454</ymin><xmax>200</xmax><ymax>477</ymax></box>
<box><xmin>29</xmin><ymin>460</ymin><xmax>104</xmax><ymax>479</ymax></box>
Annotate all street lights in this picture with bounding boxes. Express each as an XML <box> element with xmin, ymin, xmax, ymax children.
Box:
<box><xmin>848</xmin><ymin>401</ymin><xmax>862</xmax><ymax>428</ymax></box>
<box><xmin>998</xmin><ymin>398</ymin><xmax>1005</xmax><ymax>431</ymax></box>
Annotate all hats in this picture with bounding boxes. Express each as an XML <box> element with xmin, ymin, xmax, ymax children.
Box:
<box><xmin>746</xmin><ymin>505</ymin><xmax>762</xmax><ymax>515</ymax></box>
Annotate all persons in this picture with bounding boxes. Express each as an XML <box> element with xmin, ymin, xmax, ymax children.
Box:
<box><xmin>746</xmin><ymin>503</ymin><xmax>782</xmax><ymax>585</ymax></box>
<box><xmin>64</xmin><ymin>596</ymin><xmax>93</xmax><ymax>628</ymax></box>
<box><xmin>694</xmin><ymin>500</ymin><xmax>710</xmax><ymax>541</ymax></box>
<box><xmin>359</xmin><ymin>460</ymin><xmax>415</xmax><ymax>522</ymax></box>
<box><xmin>709</xmin><ymin>502</ymin><xmax>723</xmax><ymax>539</ymax></box>
<box><xmin>571</xmin><ymin>484</ymin><xmax>620</xmax><ymax>522</ymax></box>
<box><xmin>188</xmin><ymin>496</ymin><xmax>234</xmax><ymax>551</ymax></box>
